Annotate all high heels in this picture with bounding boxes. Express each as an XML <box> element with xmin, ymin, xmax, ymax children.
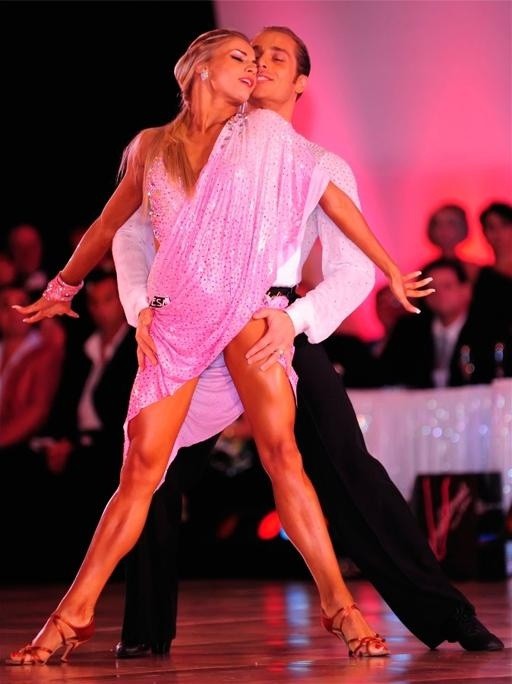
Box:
<box><xmin>320</xmin><ymin>601</ymin><xmax>391</xmax><ymax>657</ymax></box>
<box><xmin>6</xmin><ymin>616</ymin><xmax>93</xmax><ymax>669</ymax></box>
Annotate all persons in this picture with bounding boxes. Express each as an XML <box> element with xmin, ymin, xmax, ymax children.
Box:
<box><xmin>1</xmin><ymin>221</ymin><xmax>311</xmax><ymax>589</ymax></box>
<box><xmin>106</xmin><ymin>22</ymin><xmax>512</xmax><ymax>664</ymax></box>
<box><xmin>1</xmin><ymin>26</ymin><xmax>437</xmax><ymax>669</ymax></box>
<box><xmin>372</xmin><ymin>201</ymin><xmax>512</xmax><ymax>583</ymax></box>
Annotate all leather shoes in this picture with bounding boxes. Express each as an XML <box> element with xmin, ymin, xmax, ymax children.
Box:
<box><xmin>449</xmin><ymin>606</ymin><xmax>503</xmax><ymax>655</ymax></box>
<box><xmin>112</xmin><ymin>631</ymin><xmax>173</xmax><ymax>660</ymax></box>
